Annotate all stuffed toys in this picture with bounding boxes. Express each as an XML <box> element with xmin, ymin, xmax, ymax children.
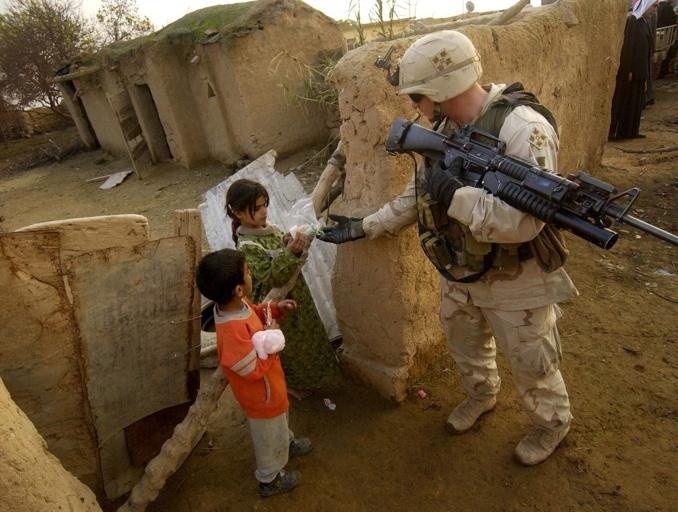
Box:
<box><xmin>251</xmin><ymin>329</ymin><xmax>286</xmax><ymax>359</ymax></box>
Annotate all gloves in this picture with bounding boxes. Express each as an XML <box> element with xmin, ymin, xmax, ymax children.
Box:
<box><xmin>425</xmin><ymin>156</ymin><xmax>463</xmax><ymax>209</ymax></box>
<box><xmin>316</xmin><ymin>215</ymin><xmax>365</xmax><ymax>244</ymax></box>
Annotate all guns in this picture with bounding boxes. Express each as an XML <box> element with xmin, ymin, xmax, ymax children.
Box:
<box><xmin>385</xmin><ymin>113</ymin><xmax>677</xmax><ymax>249</ymax></box>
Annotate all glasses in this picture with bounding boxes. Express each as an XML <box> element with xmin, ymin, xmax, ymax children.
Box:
<box><xmin>408</xmin><ymin>94</ymin><xmax>423</xmax><ymax>103</ymax></box>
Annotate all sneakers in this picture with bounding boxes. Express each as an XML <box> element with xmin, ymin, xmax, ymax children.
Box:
<box><xmin>514</xmin><ymin>423</ymin><xmax>570</xmax><ymax>466</ymax></box>
<box><xmin>288</xmin><ymin>437</ymin><xmax>313</xmax><ymax>461</ymax></box>
<box><xmin>447</xmin><ymin>394</ymin><xmax>497</xmax><ymax>434</ymax></box>
<box><xmin>260</xmin><ymin>469</ymin><xmax>301</xmax><ymax>496</ymax></box>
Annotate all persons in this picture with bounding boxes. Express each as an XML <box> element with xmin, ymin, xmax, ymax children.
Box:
<box><xmin>197</xmin><ymin>249</ymin><xmax>312</xmax><ymax>497</ymax></box>
<box><xmin>316</xmin><ymin>30</ymin><xmax>580</xmax><ymax>466</ymax></box>
<box><xmin>608</xmin><ymin>0</ymin><xmax>659</xmax><ymax>142</ymax></box>
<box><xmin>223</xmin><ymin>179</ymin><xmax>344</xmax><ymax>401</ymax></box>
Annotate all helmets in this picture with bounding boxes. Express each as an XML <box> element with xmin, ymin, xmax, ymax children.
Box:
<box><xmin>398</xmin><ymin>30</ymin><xmax>482</xmax><ymax>103</ymax></box>
